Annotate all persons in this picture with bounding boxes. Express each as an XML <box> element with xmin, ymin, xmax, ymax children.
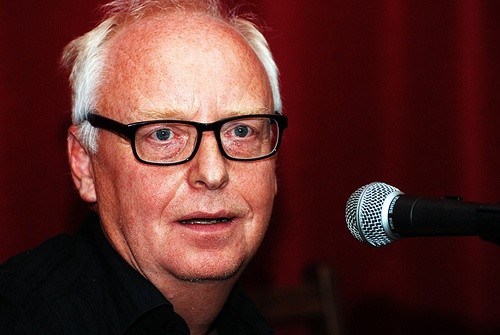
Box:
<box><xmin>0</xmin><ymin>0</ymin><xmax>294</xmax><ymax>335</ymax></box>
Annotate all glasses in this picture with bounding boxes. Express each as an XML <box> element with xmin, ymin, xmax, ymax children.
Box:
<box><xmin>87</xmin><ymin>112</ymin><xmax>288</xmax><ymax>166</ymax></box>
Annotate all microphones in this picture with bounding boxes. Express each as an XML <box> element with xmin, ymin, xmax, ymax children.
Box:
<box><xmin>345</xmin><ymin>181</ymin><xmax>500</xmax><ymax>248</ymax></box>
